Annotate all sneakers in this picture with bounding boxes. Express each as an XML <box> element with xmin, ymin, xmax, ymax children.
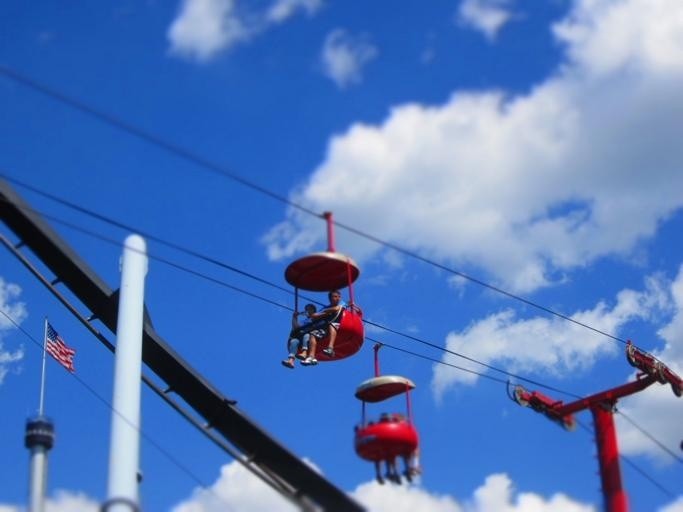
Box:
<box><xmin>295</xmin><ymin>353</ymin><xmax>306</xmax><ymax>360</ymax></box>
<box><xmin>322</xmin><ymin>348</ymin><xmax>334</xmax><ymax>357</ymax></box>
<box><xmin>300</xmin><ymin>356</ymin><xmax>317</xmax><ymax>365</ymax></box>
<box><xmin>281</xmin><ymin>360</ymin><xmax>293</xmax><ymax>368</ymax></box>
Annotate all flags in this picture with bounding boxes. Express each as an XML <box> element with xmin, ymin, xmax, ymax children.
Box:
<box><xmin>43</xmin><ymin>320</ymin><xmax>76</xmax><ymax>375</ymax></box>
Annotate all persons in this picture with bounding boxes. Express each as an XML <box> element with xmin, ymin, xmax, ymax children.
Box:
<box><xmin>390</xmin><ymin>413</ymin><xmax>423</xmax><ymax>477</ymax></box>
<box><xmin>280</xmin><ymin>303</ymin><xmax>318</xmax><ymax>369</ymax></box>
<box><xmin>299</xmin><ymin>290</ymin><xmax>346</xmax><ymax>367</ymax></box>
<box><xmin>378</xmin><ymin>411</ymin><xmax>414</xmax><ymax>484</ymax></box>
<box><xmin>363</xmin><ymin>420</ymin><xmax>393</xmax><ymax>485</ymax></box>
